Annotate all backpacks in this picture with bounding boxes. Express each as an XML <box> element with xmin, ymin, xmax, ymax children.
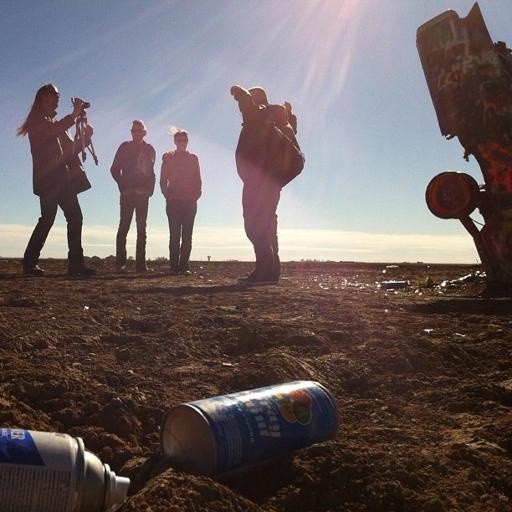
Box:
<box><xmin>255</xmin><ymin>103</ymin><xmax>305</xmax><ymax>186</ymax></box>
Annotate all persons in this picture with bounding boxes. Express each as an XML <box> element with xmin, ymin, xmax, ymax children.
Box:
<box><xmin>229</xmin><ymin>84</ymin><xmax>306</xmax><ymax>286</ymax></box>
<box><xmin>109</xmin><ymin>119</ymin><xmax>157</xmax><ymax>274</ymax></box>
<box><xmin>160</xmin><ymin>130</ymin><xmax>202</xmax><ymax>275</ymax></box>
<box><xmin>16</xmin><ymin>83</ymin><xmax>98</xmax><ymax>277</ymax></box>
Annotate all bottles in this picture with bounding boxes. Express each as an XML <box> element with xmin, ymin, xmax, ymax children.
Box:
<box><xmin>0</xmin><ymin>426</ymin><xmax>131</xmax><ymax>512</ymax></box>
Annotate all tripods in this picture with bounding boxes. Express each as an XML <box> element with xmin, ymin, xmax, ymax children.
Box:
<box><xmin>69</xmin><ymin>116</ymin><xmax>99</xmax><ymax>166</ymax></box>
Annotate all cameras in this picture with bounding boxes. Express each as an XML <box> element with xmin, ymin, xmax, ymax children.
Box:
<box><xmin>71</xmin><ymin>97</ymin><xmax>90</xmax><ymax>116</ymax></box>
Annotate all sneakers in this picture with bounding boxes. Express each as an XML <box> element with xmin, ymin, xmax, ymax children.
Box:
<box><xmin>68</xmin><ymin>263</ymin><xmax>94</xmax><ymax>277</ymax></box>
<box><xmin>115</xmin><ymin>263</ymin><xmax>154</xmax><ymax>274</ymax></box>
<box><xmin>171</xmin><ymin>265</ymin><xmax>192</xmax><ymax>275</ymax></box>
<box><xmin>21</xmin><ymin>263</ymin><xmax>45</xmax><ymax>276</ymax></box>
<box><xmin>238</xmin><ymin>272</ymin><xmax>280</xmax><ymax>284</ymax></box>
